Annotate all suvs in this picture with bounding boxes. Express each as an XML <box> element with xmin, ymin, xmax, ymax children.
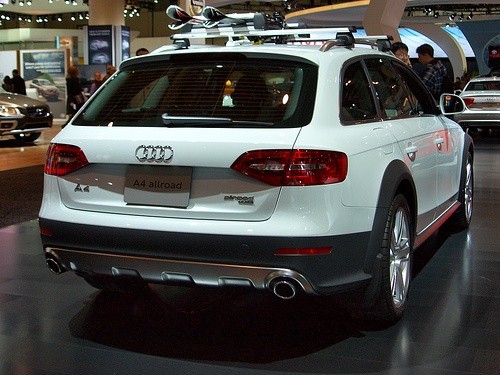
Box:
<box><xmin>37</xmin><ymin>25</ymin><xmax>476</xmax><ymax>339</ymax></box>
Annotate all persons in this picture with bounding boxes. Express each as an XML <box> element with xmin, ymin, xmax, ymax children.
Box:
<box><xmin>231</xmin><ymin>75</ymin><xmax>267</xmax><ymax>111</ymax></box>
<box><xmin>416</xmin><ymin>44</ymin><xmax>447</xmax><ymax>96</ymax></box>
<box><xmin>391</xmin><ymin>42</ymin><xmax>409</xmax><ymax>65</ymax></box>
<box><xmin>1</xmin><ymin>48</ymin><xmax>149</xmax><ymax>128</ymax></box>
<box><xmin>454</xmin><ymin>76</ymin><xmax>461</xmax><ymax>90</ymax></box>
<box><xmin>342</xmin><ymin>85</ymin><xmax>358</xmax><ymax>121</ymax></box>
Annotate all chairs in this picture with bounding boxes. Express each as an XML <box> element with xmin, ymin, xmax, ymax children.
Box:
<box><xmin>231</xmin><ymin>73</ymin><xmax>392</xmax><ymax>108</ymax></box>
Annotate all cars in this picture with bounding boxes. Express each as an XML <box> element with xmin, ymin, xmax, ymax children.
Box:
<box><xmin>29</xmin><ymin>79</ymin><xmax>60</xmax><ymax>102</ymax></box>
<box><xmin>452</xmin><ymin>76</ymin><xmax>500</xmax><ymax>133</ymax></box>
<box><xmin>92</xmin><ymin>53</ymin><xmax>110</xmax><ymax>65</ymax></box>
<box><xmin>1</xmin><ymin>85</ymin><xmax>54</xmax><ymax>142</ymax></box>
